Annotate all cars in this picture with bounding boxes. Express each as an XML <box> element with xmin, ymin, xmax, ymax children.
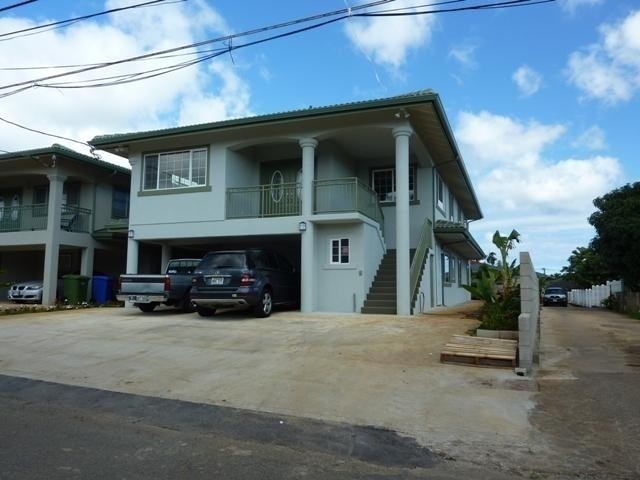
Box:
<box><xmin>542</xmin><ymin>287</ymin><xmax>568</xmax><ymax>306</ymax></box>
<box><xmin>7</xmin><ymin>271</ymin><xmax>80</xmax><ymax>303</ymax></box>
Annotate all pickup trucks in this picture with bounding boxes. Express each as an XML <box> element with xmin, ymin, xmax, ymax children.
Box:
<box><xmin>114</xmin><ymin>258</ymin><xmax>202</xmax><ymax>313</ymax></box>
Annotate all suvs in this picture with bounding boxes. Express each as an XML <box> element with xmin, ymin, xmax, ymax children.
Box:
<box><xmin>190</xmin><ymin>249</ymin><xmax>297</xmax><ymax>317</ymax></box>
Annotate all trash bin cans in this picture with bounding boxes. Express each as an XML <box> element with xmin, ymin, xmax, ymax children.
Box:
<box><xmin>62</xmin><ymin>275</ymin><xmax>113</xmax><ymax>304</ymax></box>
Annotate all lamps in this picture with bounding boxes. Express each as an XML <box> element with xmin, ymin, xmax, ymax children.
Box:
<box><xmin>299</xmin><ymin>222</ymin><xmax>305</xmax><ymax>230</ymax></box>
<box><xmin>394</xmin><ymin>107</ymin><xmax>410</xmax><ymax>119</ymax></box>
<box><xmin>128</xmin><ymin>229</ymin><xmax>134</xmax><ymax>238</ymax></box>
